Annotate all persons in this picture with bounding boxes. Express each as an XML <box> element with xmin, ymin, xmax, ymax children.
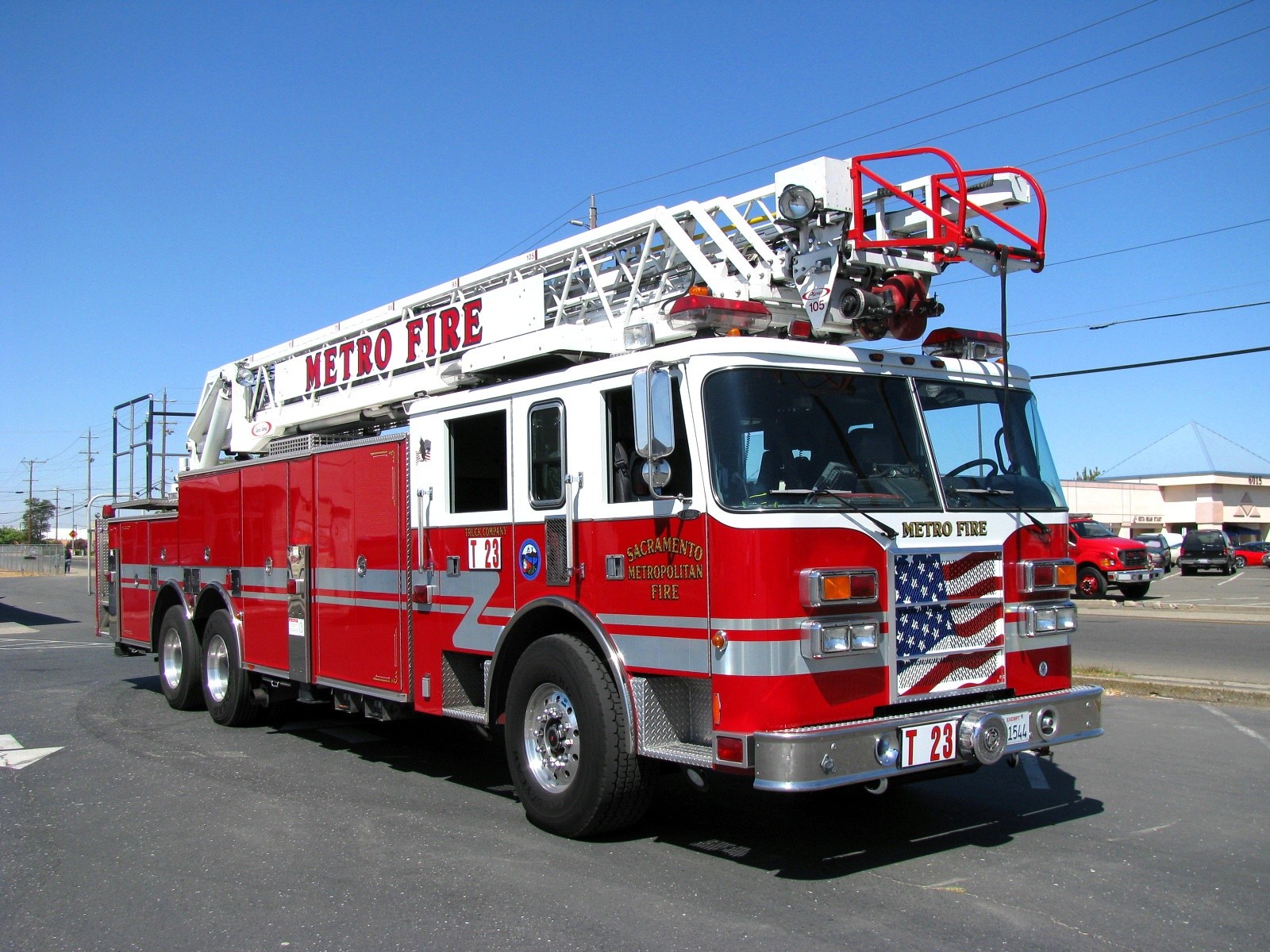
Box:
<box><xmin>64</xmin><ymin>548</ymin><xmax>72</xmax><ymax>573</ymax></box>
<box><xmin>52</xmin><ymin>552</ymin><xmax>61</xmax><ymax>574</ymax></box>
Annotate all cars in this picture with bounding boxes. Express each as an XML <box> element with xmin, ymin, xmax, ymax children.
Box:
<box><xmin>1131</xmin><ymin>533</ymin><xmax>1171</xmax><ymax>574</ymax></box>
<box><xmin>1234</xmin><ymin>541</ymin><xmax>1270</xmax><ymax>568</ymax></box>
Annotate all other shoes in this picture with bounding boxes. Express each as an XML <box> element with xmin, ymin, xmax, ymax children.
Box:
<box><xmin>65</xmin><ymin>571</ymin><xmax>67</xmax><ymax>573</ymax></box>
<box><xmin>68</xmin><ymin>571</ymin><xmax>70</xmax><ymax>573</ymax></box>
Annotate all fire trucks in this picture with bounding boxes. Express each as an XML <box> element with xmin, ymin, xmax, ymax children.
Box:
<box><xmin>87</xmin><ymin>146</ymin><xmax>1105</xmax><ymax>840</ymax></box>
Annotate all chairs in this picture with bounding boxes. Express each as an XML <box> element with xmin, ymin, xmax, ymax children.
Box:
<box><xmin>1193</xmin><ymin>538</ymin><xmax>1224</xmax><ymax>549</ymax></box>
<box><xmin>759</xmin><ymin>419</ymin><xmax>899</xmax><ymax>501</ymax></box>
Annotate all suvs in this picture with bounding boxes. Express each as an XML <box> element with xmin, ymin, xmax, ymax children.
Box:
<box><xmin>1180</xmin><ymin>529</ymin><xmax>1237</xmax><ymax>575</ymax></box>
<box><xmin>1140</xmin><ymin>532</ymin><xmax>1184</xmax><ymax>567</ymax></box>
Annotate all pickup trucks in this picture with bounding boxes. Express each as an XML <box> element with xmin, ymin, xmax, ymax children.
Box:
<box><xmin>1068</xmin><ymin>513</ymin><xmax>1161</xmax><ymax>599</ymax></box>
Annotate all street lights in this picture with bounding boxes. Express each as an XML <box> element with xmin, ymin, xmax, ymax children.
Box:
<box><xmin>52</xmin><ymin>487</ymin><xmax>75</xmax><ymax>556</ymax></box>
